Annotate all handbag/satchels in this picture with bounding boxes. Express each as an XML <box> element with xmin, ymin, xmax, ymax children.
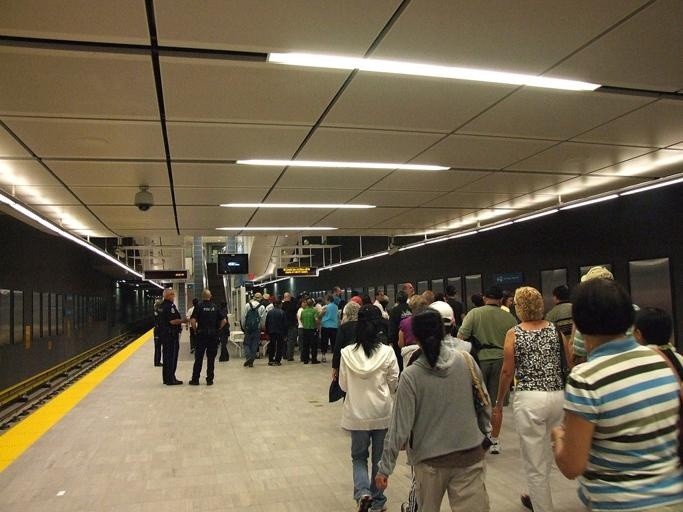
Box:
<box><xmin>464</xmin><ymin>335</ymin><xmax>482</xmax><ymax>355</ymax></box>
<box><xmin>472</xmin><ymin>377</ymin><xmax>489</xmax><ymax>412</ymax></box>
<box><xmin>329</xmin><ymin>376</ymin><xmax>345</xmax><ymax>402</ymax></box>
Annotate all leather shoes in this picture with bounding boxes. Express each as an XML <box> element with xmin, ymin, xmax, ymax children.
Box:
<box><xmin>162</xmin><ymin>378</ymin><xmax>212</xmax><ymax>386</ymax></box>
<box><xmin>154</xmin><ymin>360</ymin><xmax>164</xmax><ymax>366</ymax></box>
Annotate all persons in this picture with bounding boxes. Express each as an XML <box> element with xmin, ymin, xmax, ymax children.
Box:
<box><xmin>339</xmin><ymin>304</ymin><xmax>399</xmax><ymax>511</ymax></box>
<box><xmin>553</xmin><ymin>279</ymin><xmax>682</xmax><ymax>510</ymax></box>
<box><xmin>496</xmin><ymin>286</ymin><xmax>571</xmax><ymax>512</ymax></box>
<box><xmin>186</xmin><ymin>289</ymin><xmax>230</xmax><ymax>385</ymax></box>
<box><xmin>457</xmin><ymin>283</ymin><xmax>518</xmax><ymax>455</ymax></box>
<box><xmin>374</xmin><ymin>308</ymin><xmax>492</xmax><ymax>510</ymax></box>
<box><xmin>634</xmin><ymin>307</ymin><xmax>683</xmax><ymax>411</ymax></box>
<box><xmin>573</xmin><ymin>264</ymin><xmax>642</xmax><ymax>361</ymax></box>
<box><xmin>158</xmin><ymin>287</ymin><xmax>190</xmax><ymax>385</ymax></box>
<box><xmin>153</xmin><ymin>299</ymin><xmax>163</xmax><ymax>365</ymax></box>
<box><xmin>240</xmin><ymin>282</ymin><xmax>573</xmax><ymax>375</ymax></box>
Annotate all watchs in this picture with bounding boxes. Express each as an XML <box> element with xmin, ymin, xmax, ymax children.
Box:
<box><xmin>495</xmin><ymin>400</ymin><xmax>504</xmax><ymax>409</ymax></box>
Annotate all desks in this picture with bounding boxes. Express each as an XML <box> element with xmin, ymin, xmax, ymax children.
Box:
<box><xmin>230</xmin><ymin>331</ymin><xmax>270</xmax><ymax>359</ymax></box>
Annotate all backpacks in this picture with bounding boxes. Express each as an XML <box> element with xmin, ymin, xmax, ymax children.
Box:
<box><xmin>245</xmin><ymin>302</ymin><xmax>262</xmax><ymax>333</ymax></box>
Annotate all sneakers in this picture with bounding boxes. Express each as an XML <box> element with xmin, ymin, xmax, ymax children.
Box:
<box><xmin>358</xmin><ymin>493</ymin><xmax>410</xmax><ymax>512</ymax></box>
<box><xmin>521</xmin><ymin>494</ymin><xmax>535</xmax><ymax>510</ymax></box>
<box><xmin>245</xmin><ymin>352</ymin><xmax>326</xmax><ymax>366</ymax></box>
<box><xmin>489</xmin><ymin>437</ymin><xmax>502</xmax><ymax>454</ymax></box>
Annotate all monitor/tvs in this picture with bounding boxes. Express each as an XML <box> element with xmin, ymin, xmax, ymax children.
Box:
<box><xmin>217</xmin><ymin>253</ymin><xmax>249</xmax><ymax>275</ymax></box>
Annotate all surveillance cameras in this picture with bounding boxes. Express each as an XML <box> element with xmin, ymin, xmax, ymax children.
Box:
<box><xmin>133</xmin><ymin>189</ymin><xmax>154</xmax><ymax>212</ymax></box>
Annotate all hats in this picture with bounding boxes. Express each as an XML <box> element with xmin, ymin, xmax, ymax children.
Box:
<box><xmin>446</xmin><ymin>286</ymin><xmax>457</xmax><ymax>293</ymax></box>
<box><xmin>429</xmin><ymin>300</ymin><xmax>454</xmax><ymax>326</ymax></box>
<box><xmin>484</xmin><ymin>284</ymin><xmax>504</xmax><ymax>299</ymax></box>
<box><xmin>358</xmin><ymin>305</ymin><xmax>382</xmax><ymax>321</ymax></box>
<box><xmin>581</xmin><ymin>266</ymin><xmax>614</xmax><ymax>283</ymax></box>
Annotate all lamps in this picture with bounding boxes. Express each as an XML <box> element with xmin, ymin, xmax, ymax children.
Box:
<box><xmin>134</xmin><ymin>186</ymin><xmax>153</xmax><ymax>211</ymax></box>
<box><xmin>1</xmin><ymin>188</ymin><xmax>165</xmax><ymax>290</ymax></box>
